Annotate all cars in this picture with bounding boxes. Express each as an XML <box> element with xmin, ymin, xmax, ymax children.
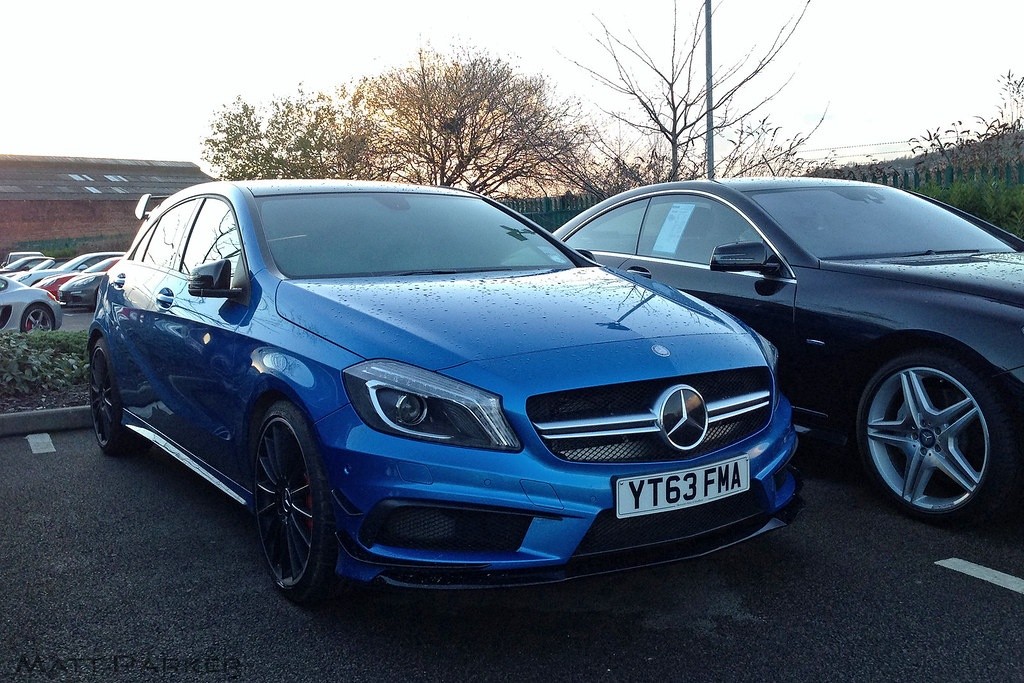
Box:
<box><xmin>85</xmin><ymin>178</ymin><xmax>809</xmax><ymax>608</ymax></box>
<box><xmin>0</xmin><ymin>251</ymin><xmax>127</xmax><ymax>337</ymax></box>
<box><xmin>541</xmin><ymin>175</ymin><xmax>1023</xmax><ymax>531</ymax></box>
